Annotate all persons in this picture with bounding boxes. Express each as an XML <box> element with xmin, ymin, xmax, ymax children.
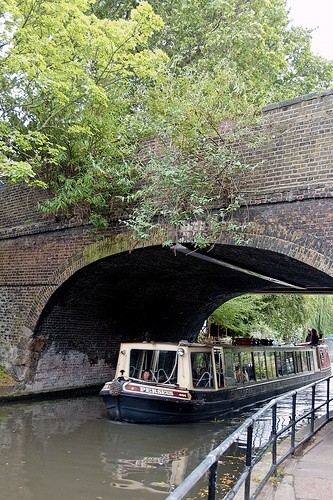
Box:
<box><xmin>142</xmin><ymin>369</ymin><xmax>152</xmax><ymax>382</ymax></box>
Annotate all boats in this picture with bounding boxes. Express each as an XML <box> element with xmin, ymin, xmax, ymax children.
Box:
<box><xmin>99</xmin><ymin>335</ymin><xmax>333</xmax><ymax>425</ymax></box>
